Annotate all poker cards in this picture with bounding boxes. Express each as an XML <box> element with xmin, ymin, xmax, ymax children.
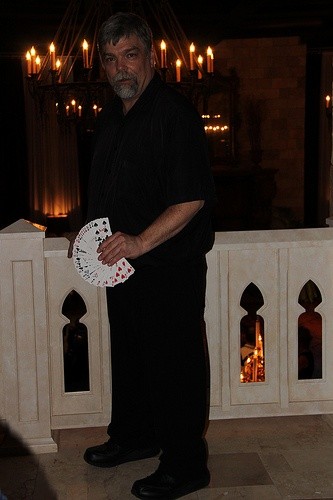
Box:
<box><xmin>72</xmin><ymin>217</ymin><xmax>136</xmax><ymax>287</ymax></box>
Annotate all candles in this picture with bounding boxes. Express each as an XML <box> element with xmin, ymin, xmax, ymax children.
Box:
<box><xmin>325</xmin><ymin>95</ymin><xmax>330</xmax><ymax>108</ymax></box>
<box><xmin>55</xmin><ymin>98</ymin><xmax>229</xmax><ymax>144</ymax></box>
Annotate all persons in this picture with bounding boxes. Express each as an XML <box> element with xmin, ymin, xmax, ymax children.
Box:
<box><xmin>66</xmin><ymin>11</ymin><xmax>214</xmax><ymax>500</ymax></box>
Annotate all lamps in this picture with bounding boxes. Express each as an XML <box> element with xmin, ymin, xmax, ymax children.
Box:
<box><xmin>24</xmin><ymin>40</ymin><xmax>214</xmax><ymax>83</ymax></box>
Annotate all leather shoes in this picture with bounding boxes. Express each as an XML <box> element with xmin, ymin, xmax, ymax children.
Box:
<box><xmin>131</xmin><ymin>465</ymin><xmax>210</xmax><ymax>500</ymax></box>
<box><xmin>84</xmin><ymin>438</ymin><xmax>159</xmax><ymax>467</ymax></box>
